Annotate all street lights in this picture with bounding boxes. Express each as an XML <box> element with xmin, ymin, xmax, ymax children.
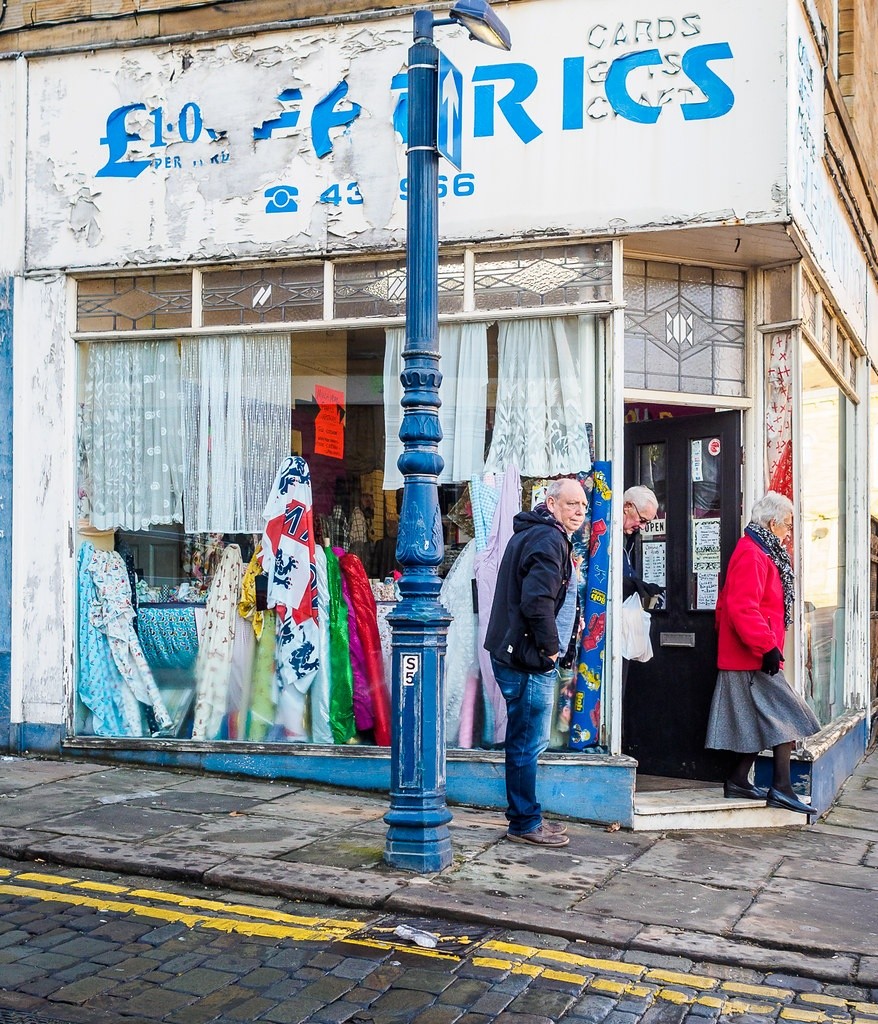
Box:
<box><xmin>367</xmin><ymin>0</ymin><xmax>513</xmax><ymax>874</ymax></box>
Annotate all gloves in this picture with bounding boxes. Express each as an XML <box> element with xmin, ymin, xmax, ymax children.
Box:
<box><xmin>762</xmin><ymin>647</ymin><xmax>785</xmax><ymax>676</ymax></box>
<box><xmin>623</xmin><ymin>576</ymin><xmax>664</xmax><ymax>598</ymax></box>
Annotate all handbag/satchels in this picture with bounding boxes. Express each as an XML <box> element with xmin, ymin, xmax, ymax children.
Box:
<box><xmin>622</xmin><ymin>592</ymin><xmax>653</xmax><ymax>662</ymax></box>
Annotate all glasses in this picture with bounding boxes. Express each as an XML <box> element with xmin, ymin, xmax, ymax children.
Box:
<box><xmin>633</xmin><ymin>503</ymin><xmax>651</xmax><ymax>525</ymax></box>
<box><xmin>785</xmin><ymin>523</ymin><xmax>792</xmax><ymax>531</ymax></box>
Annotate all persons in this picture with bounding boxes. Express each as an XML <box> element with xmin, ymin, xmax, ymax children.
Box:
<box><xmin>706</xmin><ymin>489</ymin><xmax>823</xmax><ymax>815</ymax></box>
<box><xmin>484</xmin><ymin>476</ymin><xmax>588</xmax><ymax>848</ymax></box>
<box><xmin>622</xmin><ymin>485</ymin><xmax>665</xmax><ymax>710</ymax></box>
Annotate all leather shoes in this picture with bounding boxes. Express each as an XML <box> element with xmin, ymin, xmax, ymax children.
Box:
<box><xmin>767</xmin><ymin>787</ymin><xmax>818</xmax><ymax>815</ymax></box>
<box><xmin>507</xmin><ymin>817</ymin><xmax>569</xmax><ymax>847</ymax></box>
<box><xmin>724</xmin><ymin>778</ymin><xmax>768</xmax><ymax>800</ymax></box>
